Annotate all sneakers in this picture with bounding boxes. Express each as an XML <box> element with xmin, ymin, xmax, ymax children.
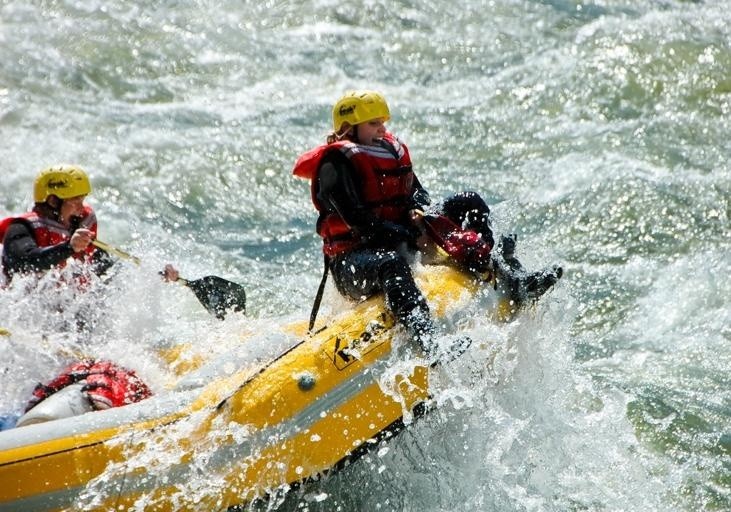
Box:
<box><xmin>428</xmin><ymin>336</ymin><xmax>472</xmax><ymax>374</ymax></box>
<box><xmin>509</xmin><ymin>264</ymin><xmax>564</xmax><ymax>309</ymax></box>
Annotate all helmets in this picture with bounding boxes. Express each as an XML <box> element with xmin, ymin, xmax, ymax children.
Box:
<box><xmin>332</xmin><ymin>90</ymin><xmax>392</xmax><ymax>135</ymax></box>
<box><xmin>32</xmin><ymin>164</ymin><xmax>93</xmax><ymax>204</ymax></box>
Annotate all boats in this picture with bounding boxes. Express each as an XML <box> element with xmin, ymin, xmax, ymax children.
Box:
<box><xmin>0</xmin><ymin>244</ymin><xmax>523</xmax><ymax>512</ymax></box>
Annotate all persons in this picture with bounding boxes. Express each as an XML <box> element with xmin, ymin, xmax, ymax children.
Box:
<box><xmin>1</xmin><ymin>163</ymin><xmax>184</xmax><ymax>344</ymax></box>
<box><xmin>312</xmin><ymin>86</ymin><xmax>566</xmax><ymax>371</ymax></box>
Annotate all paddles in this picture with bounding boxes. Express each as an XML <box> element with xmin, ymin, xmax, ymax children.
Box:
<box><xmin>91</xmin><ymin>240</ymin><xmax>245</xmax><ymax>320</ymax></box>
